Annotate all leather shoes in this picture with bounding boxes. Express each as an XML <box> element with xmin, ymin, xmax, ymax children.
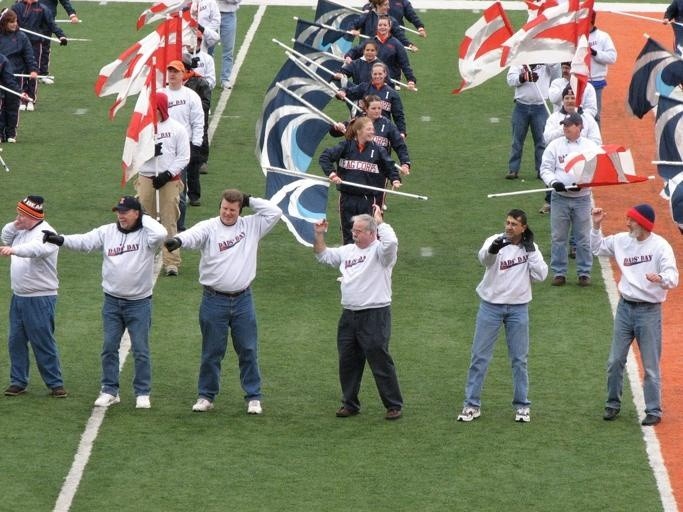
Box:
<box><xmin>385</xmin><ymin>408</ymin><xmax>402</xmax><ymax>420</ymax></box>
<box><xmin>335</xmin><ymin>406</ymin><xmax>358</xmax><ymax>417</ymax></box>
<box><xmin>603</xmin><ymin>407</ymin><xmax>620</xmax><ymax>420</ymax></box>
<box><xmin>642</xmin><ymin>414</ymin><xmax>661</xmax><ymax>426</ymax></box>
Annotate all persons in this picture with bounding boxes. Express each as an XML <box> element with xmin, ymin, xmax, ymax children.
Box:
<box><xmin>10</xmin><ymin>0</ymin><xmax>68</xmax><ymax>111</ymax></box>
<box><xmin>179</xmin><ymin>0</ymin><xmax>241</xmax><ymax>207</ymax></box>
<box><xmin>0</xmin><ymin>7</ymin><xmax>39</xmax><ymax>145</ymax></box>
<box><xmin>539</xmin><ymin>61</ymin><xmax>599</xmax><ymax>214</ymax></box>
<box><xmin>349</xmin><ymin>0</ymin><xmax>419</xmax><ymax>54</ymax></box>
<box><xmin>589</xmin><ymin>205</ymin><xmax>679</xmax><ymax>426</ymax></box>
<box><xmin>587</xmin><ymin>10</ymin><xmax>617</xmax><ymax>132</ymax></box>
<box><xmin>504</xmin><ymin>64</ymin><xmax>549</xmax><ymax>180</ymax></box>
<box><xmin>362</xmin><ymin>0</ymin><xmax>426</xmax><ymax>37</ymax></box>
<box><xmin>456</xmin><ymin>208</ymin><xmax>548</xmax><ymax>423</ymax></box>
<box><xmin>538</xmin><ymin>112</ymin><xmax>606</xmax><ymax>289</ymax></box>
<box><xmin>39</xmin><ymin>0</ymin><xmax>80</xmax><ymax>86</ymax></box>
<box><xmin>336</xmin><ymin>61</ymin><xmax>407</xmax><ymax>140</ymax></box>
<box><xmin>163</xmin><ymin>189</ymin><xmax>282</xmax><ymax>414</ymax></box>
<box><xmin>0</xmin><ymin>54</ymin><xmax>30</xmax><ymax>159</ymax></box>
<box><xmin>0</xmin><ymin>193</ymin><xmax>68</xmax><ymax>400</ymax></box>
<box><xmin>662</xmin><ymin>0</ymin><xmax>683</xmax><ymax>52</ymax></box>
<box><xmin>314</xmin><ymin>203</ymin><xmax>405</xmax><ymax>420</ymax></box>
<box><xmin>337</xmin><ymin>94</ymin><xmax>411</xmax><ymax>176</ymax></box>
<box><xmin>335</xmin><ymin>41</ymin><xmax>396</xmax><ymax>92</ymax></box>
<box><xmin>155</xmin><ymin>61</ymin><xmax>205</xmax><ymax>234</ymax></box>
<box><xmin>318</xmin><ymin>118</ymin><xmax>404</xmax><ymax>245</ymax></box>
<box><xmin>344</xmin><ymin>15</ymin><xmax>417</xmax><ymax>92</ymax></box>
<box><xmin>133</xmin><ymin>90</ymin><xmax>184</xmax><ymax>277</ymax></box>
<box><xmin>542</xmin><ymin>88</ymin><xmax>604</xmax><ymax>259</ymax></box>
<box><xmin>39</xmin><ymin>195</ymin><xmax>168</xmax><ymax>410</ymax></box>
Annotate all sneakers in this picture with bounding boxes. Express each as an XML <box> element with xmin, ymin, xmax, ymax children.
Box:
<box><xmin>199</xmin><ymin>162</ymin><xmax>208</xmax><ymax>174</ymax></box>
<box><xmin>539</xmin><ymin>203</ymin><xmax>550</xmax><ymax>213</ymax></box>
<box><xmin>551</xmin><ymin>276</ymin><xmax>566</xmax><ymax>286</ymax></box>
<box><xmin>4</xmin><ymin>384</ymin><xmax>27</xmax><ymax>395</ymax></box>
<box><xmin>6</xmin><ymin>133</ymin><xmax>16</xmax><ymax>143</ymax></box>
<box><xmin>166</xmin><ymin>265</ymin><xmax>178</xmax><ymax>276</ymax></box>
<box><xmin>52</xmin><ymin>386</ymin><xmax>67</xmax><ymax>398</ymax></box>
<box><xmin>190</xmin><ymin>198</ymin><xmax>200</xmax><ymax>206</ymax></box>
<box><xmin>94</xmin><ymin>390</ymin><xmax>121</xmax><ymax>406</ymax></box>
<box><xmin>27</xmin><ymin>102</ymin><xmax>34</xmax><ymax>111</ymax></box>
<box><xmin>456</xmin><ymin>405</ymin><xmax>481</xmax><ymax>422</ymax></box>
<box><xmin>19</xmin><ymin>104</ymin><xmax>27</xmax><ymax>111</ymax></box>
<box><xmin>39</xmin><ymin>78</ymin><xmax>55</xmax><ymax>85</ymax></box>
<box><xmin>505</xmin><ymin>171</ymin><xmax>517</xmax><ymax>180</ymax></box>
<box><xmin>136</xmin><ymin>395</ymin><xmax>152</xmax><ymax>409</ymax></box>
<box><xmin>192</xmin><ymin>398</ymin><xmax>214</xmax><ymax>412</ymax></box>
<box><xmin>220</xmin><ymin>82</ymin><xmax>232</xmax><ymax>89</ymax></box>
<box><xmin>247</xmin><ymin>400</ymin><xmax>262</xmax><ymax>414</ymax></box>
<box><xmin>579</xmin><ymin>276</ymin><xmax>591</xmax><ymax>286</ymax></box>
<box><xmin>515</xmin><ymin>406</ymin><xmax>531</xmax><ymax>423</ymax></box>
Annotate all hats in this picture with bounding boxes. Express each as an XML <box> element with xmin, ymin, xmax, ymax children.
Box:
<box><xmin>626</xmin><ymin>204</ymin><xmax>655</xmax><ymax>232</ymax></box>
<box><xmin>562</xmin><ymin>87</ymin><xmax>574</xmax><ymax>97</ymax></box>
<box><xmin>16</xmin><ymin>196</ymin><xmax>44</xmax><ymax>221</ymax></box>
<box><xmin>112</xmin><ymin>195</ymin><xmax>140</xmax><ymax>211</ymax></box>
<box><xmin>166</xmin><ymin>60</ymin><xmax>186</xmax><ymax>72</ymax></box>
<box><xmin>559</xmin><ymin>112</ymin><xmax>583</xmax><ymax>125</ymax></box>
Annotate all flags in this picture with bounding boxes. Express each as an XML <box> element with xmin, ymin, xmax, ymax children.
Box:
<box><xmin>655</xmin><ymin>159</ymin><xmax>683</xmax><ymax>235</ymax></box>
<box><xmin>109</xmin><ymin>20</ymin><xmax>169</xmax><ymax>119</ymax></box>
<box><xmin>451</xmin><ymin>0</ymin><xmax>514</xmax><ymax>95</ymax></box>
<box><xmin>136</xmin><ymin>0</ymin><xmax>193</xmax><ymax>32</ymax></box>
<box><xmin>314</xmin><ymin>0</ymin><xmax>363</xmax><ymax>32</ymax></box>
<box><xmin>95</xmin><ymin>15</ymin><xmax>183</xmax><ymax>99</ymax></box>
<box><xmin>499</xmin><ymin>0</ymin><xmax>580</xmax><ymax>68</ymax></box>
<box><xmin>654</xmin><ymin>93</ymin><xmax>683</xmax><ymax>201</ymax></box>
<box><xmin>292</xmin><ymin>41</ymin><xmax>345</xmax><ymax>84</ymax></box>
<box><xmin>253</xmin><ymin>82</ymin><xmax>336</xmax><ymax>177</ymax></box>
<box><xmin>264</xmin><ymin>166</ymin><xmax>331</xmax><ymax>248</ymax></box>
<box><xmin>625</xmin><ymin>38</ymin><xmax>683</xmax><ymax>120</ymax></box>
<box><xmin>670</xmin><ymin>22</ymin><xmax>683</xmax><ymax>58</ymax></box>
<box><xmin>577</xmin><ymin>0</ymin><xmax>594</xmax><ymax>45</ymax></box>
<box><xmin>293</xmin><ymin>18</ymin><xmax>357</xmax><ymax>91</ymax></box>
<box><xmin>569</xmin><ymin>32</ymin><xmax>592</xmax><ymax>107</ymax></box>
<box><xmin>119</xmin><ymin>63</ymin><xmax>158</xmax><ymax>187</ymax></box>
<box><xmin>261</xmin><ymin>58</ymin><xmax>338</xmax><ymax>112</ymax></box>
<box><xmin>563</xmin><ymin>143</ymin><xmax>649</xmax><ymax>187</ymax></box>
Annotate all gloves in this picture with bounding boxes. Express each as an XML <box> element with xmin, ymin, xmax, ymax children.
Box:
<box><xmin>552</xmin><ymin>182</ymin><xmax>567</xmax><ymax>193</ymax></box>
<box><xmin>155</xmin><ymin>142</ymin><xmax>163</xmax><ymax>157</ymax></box>
<box><xmin>42</xmin><ymin>228</ymin><xmax>64</xmax><ymax>247</ymax></box>
<box><xmin>152</xmin><ymin>170</ymin><xmax>172</xmax><ymax>190</ymax></box>
<box><xmin>568</xmin><ymin>183</ymin><xmax>580</xmax><ymax>191</ymax></box>
<box><xmin>242</xmin><ymin>193</ymin><xmax>250</xmax><ymax>208</ymax></box>
<box><xmin>164</xmin><ymin>237</ymin><xmax>182</xmax><ymax>253</ymax></box>
<box><xmin>521</xmin><ymin>227</ymin><xmax>535</xmax><ymax>252</ymax></box>
<box><xmin>487</xmin><ymin>236</ymin><xmax>512</xmax><ymax>254</ymax></box>
<box><xmin>518</xmin><ymin>71</ymin><xmax>539</xmax><ymax>84</ymax></box>
<box><xmin>60</xmin><ymin>39</ymin><xmax>68</xmax><ymax>46</ymax></box>
